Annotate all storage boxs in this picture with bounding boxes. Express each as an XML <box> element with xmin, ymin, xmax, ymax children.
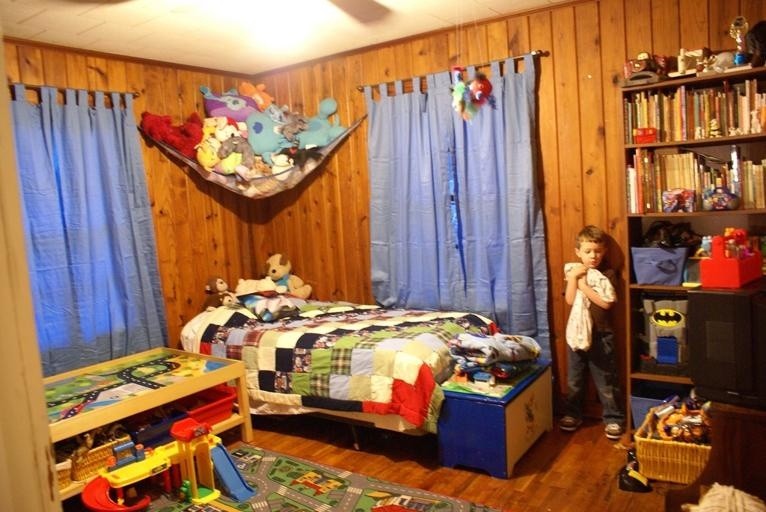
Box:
<box><xmin>629</xmin><ymin>394</ymin><xmax>683</xmax><ymax>431</ymax></box>
<box><xmin>630</xmin><ymin>245</ymin><xmax>687</xmax><ymax>287</ymax></box>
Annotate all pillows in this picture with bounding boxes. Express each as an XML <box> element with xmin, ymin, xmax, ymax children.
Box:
<box><xmin>236</xmin><ymin>289</ymin><xmax>302</xmax><ymax>322</ymax></box>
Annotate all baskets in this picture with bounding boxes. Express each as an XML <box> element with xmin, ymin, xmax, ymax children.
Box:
<box><xmin>634</xmin><ymin>407</ymin><xmax>711</xmax><ymax>484</ymax></box>
<box><xmin>56</xmin><ymin>386</ymin><xmax>236</xmax><ymax>491</ymax></box>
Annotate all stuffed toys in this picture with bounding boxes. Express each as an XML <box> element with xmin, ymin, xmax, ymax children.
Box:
<box><xmin>265</xmin><ymin>249</ymin><xmax>312</xmax><ymax>300</ymax></box>
<box><xmin>137</xmin><ymin>76</ymin><xmax>347</xmax><ymax>199</ymax></box>
<box><xmin>448</xmin><ymin>67</ymin><xmax>498</xmax><ymax>128</ymax></box>
<box><xmin>203</xmin><ymin>275</ymin><xmax>237</xmax><ymax>311</ymax></box>
<box><xmin>234</xmin><ymin>275</ymin><xmax>288</xmax><ymax>295</ymax></box>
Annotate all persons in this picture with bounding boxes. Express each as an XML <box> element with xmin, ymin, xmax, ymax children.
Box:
<box><xmin>558</xmin><ymin>222</ymin><xmax>625</xmax><ymax>441</ymax></box>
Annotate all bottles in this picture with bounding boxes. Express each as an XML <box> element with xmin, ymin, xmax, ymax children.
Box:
<box><xmin>734</xmin><ymin>29</ymin><xmax>746</xmax><ymax>66</ymax></box>
<box><xmin>712</xmin><ymin>178</ymin><xmax>730</xmax><ymax>210</ymax></box>
<box><xmin>678</xmin><ymin>48</ymin><xmax>688</xmax><ymax>73</ymax></box>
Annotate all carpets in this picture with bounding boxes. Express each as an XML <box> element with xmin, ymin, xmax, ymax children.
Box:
<box><xmin>138</xmin><ymin>443</ymin><xmax>504</xmax><ymax>512</ymax></box>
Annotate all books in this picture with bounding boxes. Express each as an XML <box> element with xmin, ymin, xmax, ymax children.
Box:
<box><xmin>624</xmin><ymin>77</ymin><xmax>765</xmax><ymax>214</ymax></box>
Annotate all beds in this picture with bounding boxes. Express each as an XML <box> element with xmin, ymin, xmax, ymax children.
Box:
<box><xmin>180</xmin><ymin>299</ymin><xmax>502</xmax><ymax>436</ymax></box>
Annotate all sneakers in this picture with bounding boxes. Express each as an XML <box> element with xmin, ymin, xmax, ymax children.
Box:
<box><xmin>560</xmin><ymin>416</ymin><xmax>583</xmax><ymax>431</ymax></box>
<box><xmin>604</xmin><ymin>424</ymin><xmax>623</xmax><ymax>439</ymax></box>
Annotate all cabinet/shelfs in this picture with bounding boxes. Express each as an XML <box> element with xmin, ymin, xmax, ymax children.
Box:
<box><xmin>665</xmin><ymin>402</ymin><xmax>766</xmax><ymax>512</ymax></box>
<box><xmin>44</xmin><ymin>346</ymin><xmax>254</xmax><ymax>501</ymax></box>
<box><xmin>616</xmin><ymin>66</ymin><xmax>766</xmax><ymax>446</ymax></box>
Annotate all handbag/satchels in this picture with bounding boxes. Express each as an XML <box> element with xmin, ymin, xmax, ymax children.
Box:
<box><xmin>702</xmin><ymin>187</ymin><xmax>739</xmax><ymax>210</ymax></box>
<box><xmin>639</xmin><ymin>220</ymin><xmax>690</xmax><ymax>247</ymax></box>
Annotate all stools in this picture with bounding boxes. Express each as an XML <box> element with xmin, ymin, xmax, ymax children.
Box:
<box><xmin>436</xmin><ymin>357</ymin><xmax>553</xmax><ymax>479</ymax></box>
